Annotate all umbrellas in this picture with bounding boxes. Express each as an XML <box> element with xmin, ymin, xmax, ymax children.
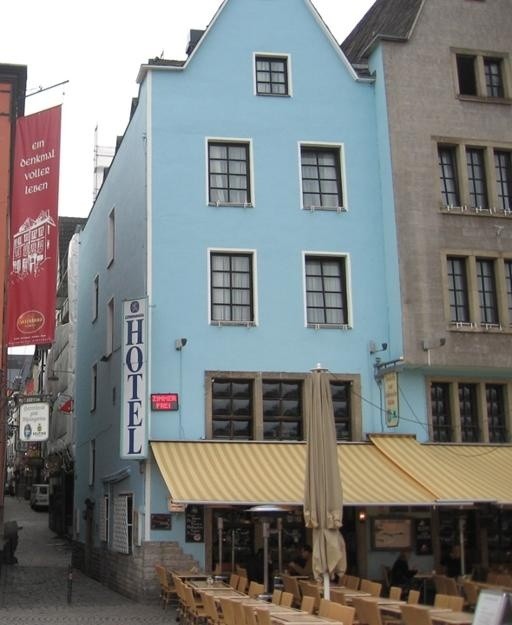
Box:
<box><xmin>297</xmin><ymin>359</ymin><xmax>349</xmax><ymax>599</ymax></box>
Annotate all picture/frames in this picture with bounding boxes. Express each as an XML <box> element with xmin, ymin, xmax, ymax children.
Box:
<box><xmin>371</xmin><ymin>516</ymin><xmax>416</xmax><ymax>553</ymax></box>
<box><xmin>151</xmin><ymin>513</ymin><xmax>172</xmax><ymax>531</ymax></box>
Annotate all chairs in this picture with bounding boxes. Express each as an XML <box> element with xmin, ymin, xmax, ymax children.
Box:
<box><xmin>156</xmin><ymin>561</ymin><xmax>512</xmax><ymax>625</ymax></box>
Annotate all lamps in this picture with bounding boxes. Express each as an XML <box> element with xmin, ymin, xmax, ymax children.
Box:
<box><xmin>358</xmin><ymin>507</ymin><xmax>367</xmax><ymax>525</ymax></box>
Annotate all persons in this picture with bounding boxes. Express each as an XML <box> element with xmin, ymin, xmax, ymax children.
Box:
<box><xmin>390</xmin><ymin>546</ymin><xmax>420</xmax><ymax>588</ymax></box>
<box><xmin>286</xmin><ymin>541</ymin><xmax>313</xmax><ymax>579</ymax></box>
<box><xmin>249</xmin><ymin>546</ymin><xmax>273</xmax><ymax>600</ymax></box>
<box><xmin>440</xmin><ymin>546</ymin><xmax>472</xmax><ymax>576</ymax></box>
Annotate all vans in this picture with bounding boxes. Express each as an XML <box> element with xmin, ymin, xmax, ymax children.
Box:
<box><xmin>30</xmin><ymin>484</ymin><xmax>49</xmax><ymax>510</ymax></box>
